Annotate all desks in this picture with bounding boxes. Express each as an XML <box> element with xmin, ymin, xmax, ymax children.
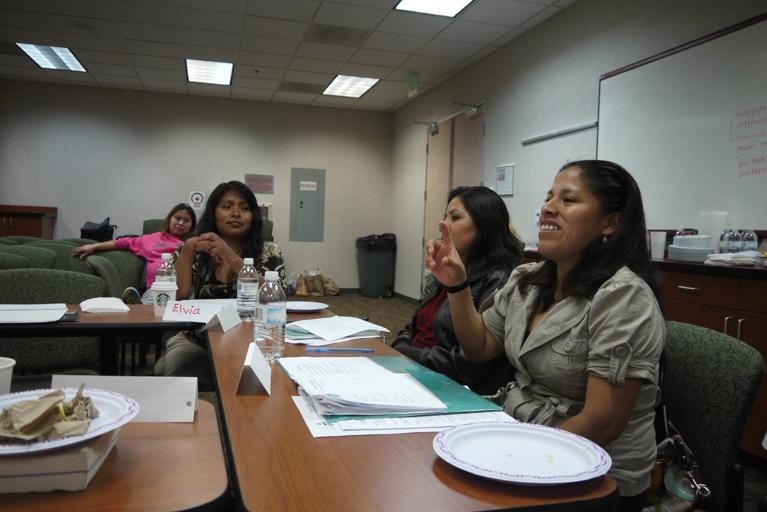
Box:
<box><xmin>0</xmin><ymin>381</ymin><xmax>227</xmax><ymax>509</ymax></box>
<box><xmin>0</xmin><ymin>298</ymin><xmax>623</xmax><ymax>510</ymax></box>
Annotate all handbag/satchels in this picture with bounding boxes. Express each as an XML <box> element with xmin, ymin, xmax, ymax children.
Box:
<box><xmin>641</xmin><ymin>435</ymin><xmax>711</xmax><ymax>512</ymax></box>
<box><xmin>304</xmin><ymin>270</ymin><xmax>325</xmax><ymax>297</ymax></box>
<box><xmin>321</xmin><ymin>273</ymin><xmax>339</xmax><ymax>296</ymax></box>
<box><xmin>295</xmin><ymin>271</ymin><xmax>310</xmax><ymax>296</ymax></box>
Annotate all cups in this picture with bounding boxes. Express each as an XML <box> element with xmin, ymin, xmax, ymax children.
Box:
<box><xmin>149</xmin><ymin>280</ymin><xmax>178</xmax><ymax>317</ymax></box>
<box><xmin>0</xmin><ymin>356</ymin><xmax>16</xmax><ymax>394</ymax></box>
<box><xmin>649</xmin><ymin>231</ymin><xmax>666</xmax><ymax>260</ymax></box>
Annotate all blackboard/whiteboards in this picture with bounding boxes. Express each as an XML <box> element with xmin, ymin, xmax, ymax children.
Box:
<box><xmin>595</xmin><ymin>11</ymin><xmax>767</xmax><ymax>238</ymax></box>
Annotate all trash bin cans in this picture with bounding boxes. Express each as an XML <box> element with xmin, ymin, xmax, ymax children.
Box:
<box><xmin>356</xmin><ymin>233</ymin><xmax>397</xmax><ymax>298</ymax></box>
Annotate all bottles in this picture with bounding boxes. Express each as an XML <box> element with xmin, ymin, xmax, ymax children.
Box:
<box><xmin>715</xmin><ymin>222</ymin><xmax>758</xmax><ymax>253</ymax></box>
<box><xmin>153</xmin><ymin>252</ymin><xmax>176</xmax><ymax>280</ymax></box>
<box><xmin>236</xmin><ymin>256</ymin><xmax>258</xmax><ymax>317</ymax></box>
<box><xmin>252</xmin><ymin>270</ymin><xmax>285</xmax><ymax>363</ymax></box>
<box><xmin>675</xmin><ymin>226</ymin><xmax>686</xmax><ymax>235</ymax></box>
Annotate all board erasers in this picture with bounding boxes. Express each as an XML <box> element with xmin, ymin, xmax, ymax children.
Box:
<box><xmin>683</xmin><ymin>228</ymin><xmax>698</xmax><ymax>235</ymax></box>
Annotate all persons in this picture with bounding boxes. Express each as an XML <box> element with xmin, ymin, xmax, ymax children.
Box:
<box><xmin>388</xmin><ymin>184</ymin><xmax>524</xmax><ymax>405</ymax></box>
<box><xmin>421</xmin><ymin>157</ymin><xmax>659</xmax><ymax>511</ymax></box>
<box><xmin>67</xmin><ymin>202</ymin><xmax>196</xmax><ymax>303</ymax></box>
<box><xmin>154</xmin><ymin>178</ymin><xmax>288</xmax><ymax>390</ymax></box>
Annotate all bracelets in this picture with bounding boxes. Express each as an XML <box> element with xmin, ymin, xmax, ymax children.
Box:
<box><xmin>442</xmin><ymin>278</ymin><xmax>473</xmax><ymax>295</ymax></box>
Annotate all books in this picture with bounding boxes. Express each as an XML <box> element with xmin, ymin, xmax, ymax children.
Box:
<box><xmin>276</xmin><ymin>351</ymin><xmax>447</xmax><ymax>416</ymax></box>
<box><xmin>78</xmin><ymin>292</ymin><xmax>131</xmax><ymax>314</ymax></box>
<box><xmin>0</xmin><ymin>441</ymin><xmax>121</xmax><ymax>497</ymax></box>
<box><xmin>0</xmin><ymin>421</ymin><xmax>121</xmax><ymax>474</ymax></box>
<box><xmin>280</xmin><ymin>314</ymin><xmax>389</xmax><ymax>344</ymax></box>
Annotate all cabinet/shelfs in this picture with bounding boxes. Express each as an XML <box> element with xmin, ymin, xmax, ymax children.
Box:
<box><xmin>644</xmin><ymin>248</ymin><xmax>767</xmax><ymax>471</ymax></box>
<box><xmin>0</xmin><ymin>204</ymin><xmax>64</xmax><ymax>244</ymax></box>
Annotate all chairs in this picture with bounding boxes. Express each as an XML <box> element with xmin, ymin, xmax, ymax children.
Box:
<box><xmin>647</xmin><ymin>316</ymin><xmax>766</xmax><ymax>510</ymax></box>
<box><xmin>0</xmin><ymin>219</ymin><xmax>272</xmax><ymax>302</ymax></box>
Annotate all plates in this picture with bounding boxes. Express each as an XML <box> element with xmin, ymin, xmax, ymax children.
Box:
<box><xmin>0</xmin><ymin>387</ymin><xmax>139</xmax><ymax>456</ymax></box>
<box><xmin>431</xmin><ymin>422</ymin><xmax>615</xmax><ymax>485</ymax></box>
<box><xmin>277</xmin><ymin>299</ymin><xmax>329</xmax><ymax>314</ymax></box>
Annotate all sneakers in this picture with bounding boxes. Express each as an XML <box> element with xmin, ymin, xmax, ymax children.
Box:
<box><xmin>122</xmin><ymin>287</ymin><xmax>141</xmax><ymax>304</ymax></box>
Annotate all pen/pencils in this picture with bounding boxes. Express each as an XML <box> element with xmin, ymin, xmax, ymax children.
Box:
<box><xmin>305</xmin><ymin>347</ymin><xmax>375</xmax><ymax>352</ymax></box>
